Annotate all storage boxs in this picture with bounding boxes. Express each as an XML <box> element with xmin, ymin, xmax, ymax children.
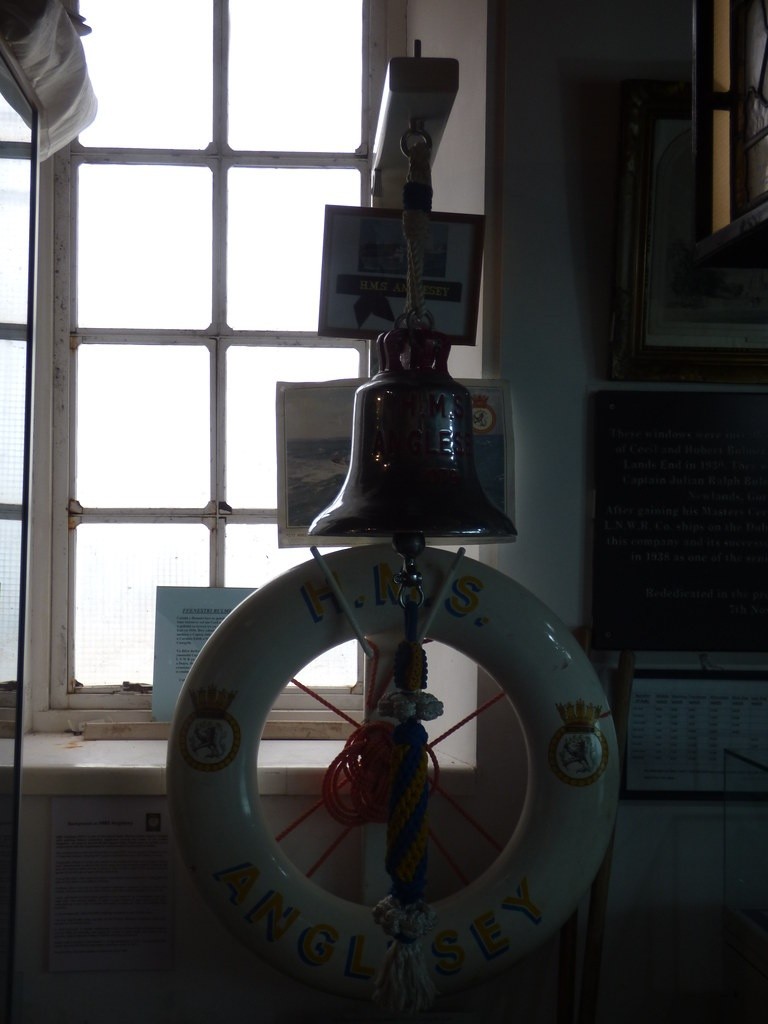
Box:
<box><xmin>623</xmin><ymin>678</ymin><xmax>768</xmax><ymax>791</ymax></box>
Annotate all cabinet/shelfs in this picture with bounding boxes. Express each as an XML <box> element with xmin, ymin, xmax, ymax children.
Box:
<box><xmin>593</xmin><ymin>800</ymin><xmax>768</xmax><ymax>1024</ymax></box>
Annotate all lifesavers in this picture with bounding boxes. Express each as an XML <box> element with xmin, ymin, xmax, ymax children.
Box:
<box><xmin>165</xmin><ymin>544</ymin><xmax>630</xmax><ymax>1008</ymax></box>
